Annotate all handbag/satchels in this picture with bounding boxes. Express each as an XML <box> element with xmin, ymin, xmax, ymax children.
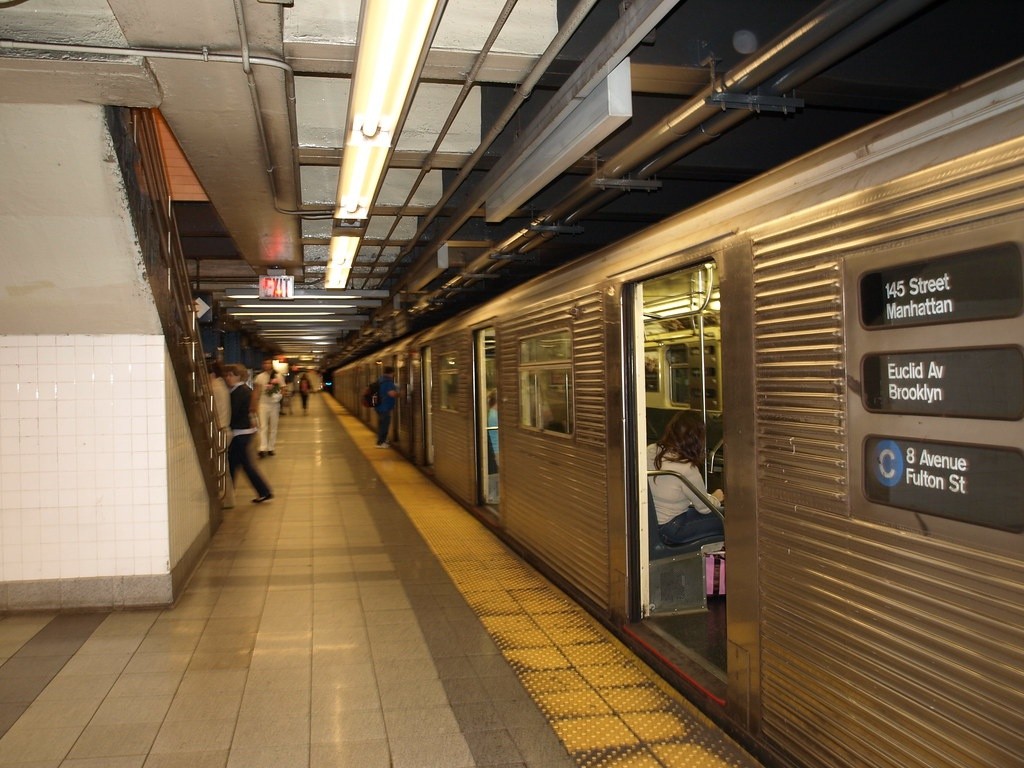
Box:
<box><xmin>703</xmin><ymin>545</ymin><xmax>724</xmax><ymax>600</ymax></box>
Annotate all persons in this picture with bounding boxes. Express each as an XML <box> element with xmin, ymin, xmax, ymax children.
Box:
<box><xmin>375</xmin><ymin>366</ymin><xmax>398</xmax><ymax>449</ymax></box>
<box><xmin>647</xmin><ymin>410</ymin><xmax>724</xmax><ymax>545</ymax></box>
<box><xmin>279</xmin><ymin>374</ymin><xmax>310</xmax><ymax>416</ymax></box>
<box><xmin>250</xmin><ymin>359</ymin><xmax>286</xmax><ymax>457</ymax></box>
<box><xmin>208</xmin><ymin>363</ymin><xmax>236</xmax><ymax>509</ymax></box>
<box><xmin>225</xmin><ymin>364</ymin><xmax>274</xmax><ymax>504</ymax></box>
<box><xmin>488</xmin><ymin>388</ymin><xmax>499</xmax><ymax>467</ymax></box>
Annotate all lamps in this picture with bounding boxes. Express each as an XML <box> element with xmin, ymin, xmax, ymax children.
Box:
<box><xmin>324</xmin><ymin>226</ymin><xmax>365</xmax><ymax>291</ymax></box>
<box><xmin>331</xmin><ymin>0</ymin><xmax>448</xmax><ymax>219</ymax></box>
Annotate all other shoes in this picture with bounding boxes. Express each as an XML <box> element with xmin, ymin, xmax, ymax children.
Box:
<box><xmin>376</xmin><ymin>442</ymin><xmax>390</xmax><ymax>449</ymax></box>
<box><xmin>252</xmin><ymin>493</ymin><xmax>273</xmax><ymax>504</ymax></box>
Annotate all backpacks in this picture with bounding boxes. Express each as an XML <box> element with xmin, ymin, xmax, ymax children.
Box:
<box><xmin>361</xmin><ymin>378</ymin><xmax>384</xmax><ymax>407</ymax></box>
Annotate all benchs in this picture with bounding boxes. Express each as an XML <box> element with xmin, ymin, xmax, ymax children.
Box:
<box><xmin>647</xmin><ymin>406</ymin><xmax>725</xmax><ymax>466</ymax></box>
<box><xmin>487</xmin><ymin>431</ymin><xmax>499</xmax><ymax>505</ymax></box>
<box><xmin>645</xmin><ymin>477</ymin><xmax>725</xmax><ymax>612</ymax></box>
